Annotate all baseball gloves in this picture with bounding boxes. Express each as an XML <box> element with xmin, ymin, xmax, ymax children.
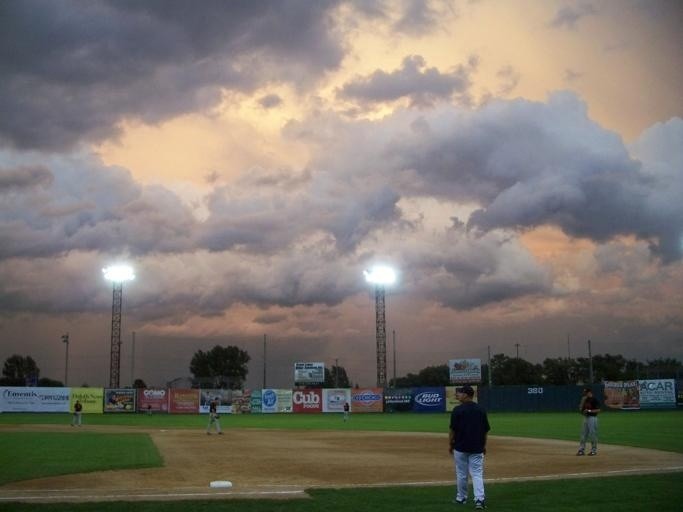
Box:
<box><xmin>584</xmin><ymin>409</ymin><xmax>590</xmax><ymax>416</ymax></box>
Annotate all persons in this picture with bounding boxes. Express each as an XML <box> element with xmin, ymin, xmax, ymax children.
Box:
<box><xmin>447</xmin><ymin>384</ymin><xmax>491</xmax><ymax>510</ymax></box>
<box><xmin>576</xmin><ymin>388</ymin><xmax>601</xmax><ymax>455</ymax></box>
<box><xmin>205</xmin><ymin>396</ymin><xmax>224</xmax><ymax>435</ymax></box>
<box><xmin>147</xmin><ymin>404</ymin><xmax>152</xmax><ymax>416</ymax></box>
<box><xmin>343</xmin><ymin>402</ymin><xmax>350</xmax><ymax>421</ymax></box>
<box><xmin>69</xmin><ymin>400</ymin><xmax>82</xmax><ymax>427</ymax></box>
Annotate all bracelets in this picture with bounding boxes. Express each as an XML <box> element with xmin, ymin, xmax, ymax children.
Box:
<box><xmin>587</xmin><ymin>409</ymin><xmax>592</xmax><ymax>413</ymax></box>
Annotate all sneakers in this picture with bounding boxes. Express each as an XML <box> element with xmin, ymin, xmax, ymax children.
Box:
<box><xmin>207</xmin><ymin>431</ymin><xmax>223</xmax><ymax>435</ymax></box>
<box><xmin>578</xmin><ymin>449</ymin><xmax>597</xmax><ymax>455</ymax></box>
<box><xmin>475</xmin><ymin>498</ymin><xmax>487</xmax><ymax>509</ymax></box>
<box><xmin>455</xmin><ymin>496</ymin><xmax>466</xmax><ymax>505</ymax></box>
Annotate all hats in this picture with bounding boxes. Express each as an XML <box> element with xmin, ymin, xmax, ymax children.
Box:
<box><xmin>583</xmin><ymin>386</ymin><xmax>591</xmax><ymax>396</ymax></box>
<box><xmin>456</xmin><ymin>385</ymin><xmax>474</xmax><ymax>396</ymax></box>
